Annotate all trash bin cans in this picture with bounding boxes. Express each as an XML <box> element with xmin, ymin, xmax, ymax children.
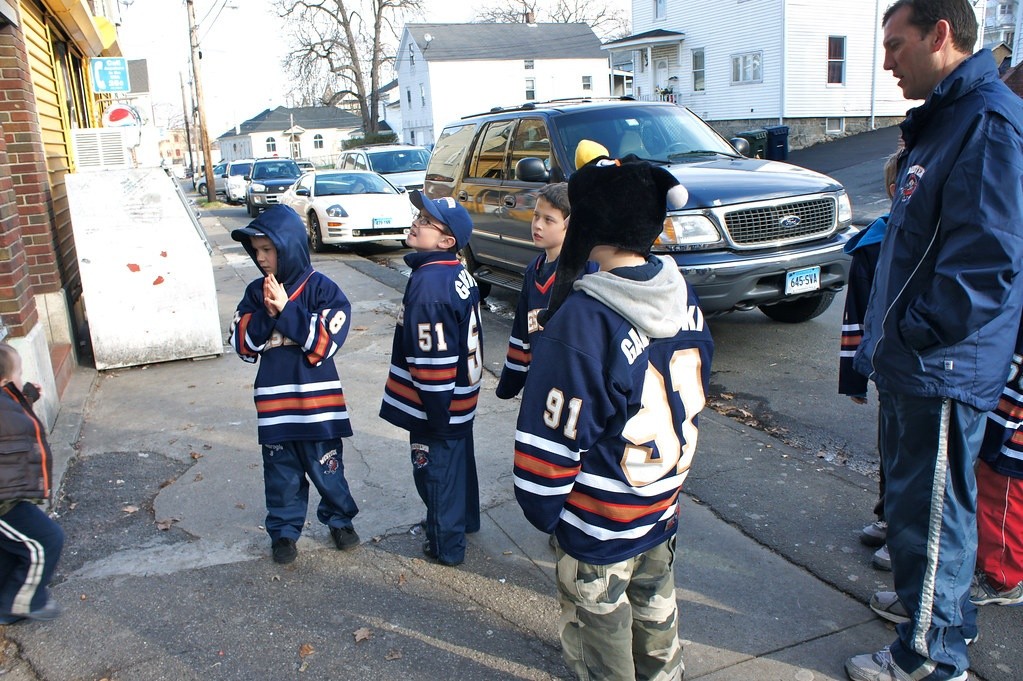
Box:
<box><xmin>735</xmin><ymin>126</ymin><xmax>790</xmax><ymax>160</ymax></box>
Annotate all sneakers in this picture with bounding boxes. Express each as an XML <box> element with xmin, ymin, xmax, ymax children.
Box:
<box><xmin>0</xmin><ymin>599</ymin><xmax>61</xmax><ymax>626</ymax></box>
<box><xmin>871</xmin><ymin>544</ymin><xmax>892</xmax><ymax>571</ymax></box>
<box><xmin>422</xmin><ymin>541</ymin><xmax>464</xmax><ymax>566</ymax></box>
<box><xmin>845</xmin><ymin>646</ymin><xmax>969</xmax><ymax>681</ymax></box>
<box><xmin>330</xmin><ymin>525</ymin><xmax>360</xmax><ymax>550</ymax></box>
<box><xmin>420</xmin><ymin>518</ymin><xmax>427</xmax><ymax>528</ymax></box>
<box><xmin>861</xmin><ymin>520</ymin><xmax>887</xmax><ymax>546</ymax></box>
<box><xmin>271</xmin><ymin>537</ymin><xmax>298</xmax><ymax>565</ymax></box>
<box><xmin>969</xmin><ymin>568</ymin><xmax>1023</xmax><ymax>606</ymax></box>
<box><xmin>870</xmin><ymin>592</ymin><xmax>979</xmax><ymax>645</ymax></box>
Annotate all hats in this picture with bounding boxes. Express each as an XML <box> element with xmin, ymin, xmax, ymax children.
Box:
<box><xmin>537</xmin><ymin>140</ymin><xmax>688</xmax><ymax>327</ymax></box>
<box><xmin>409</xmin><ymin>189</ymin><xmax>473</xmax><ymax>252</ymax></box>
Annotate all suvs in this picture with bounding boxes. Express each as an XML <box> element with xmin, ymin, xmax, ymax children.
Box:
<box><xmin>424</xmin><ymin>95</ymin><xmax>859</xmax><ymax>327</ymax></box>
<box><xmin>244</xmin><ymin>157</ymin><xmax>302</xmax><ymax>218</ymax></box>
<box><xmin>334</xmin><ymin>143</ymin><xmax>432</xmax><ymax>196</ymax></box>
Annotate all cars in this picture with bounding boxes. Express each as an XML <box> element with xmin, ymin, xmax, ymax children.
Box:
<box><xmin>280</xmin><ymin>170</ymin><xmax>419</xmax><ymax>253</ymax></box>
<box><xmin>194</xmin><ymin>163</ymin><xmax>226</xmax><ymax>196</ymax></box>
<box><xmin>222</xmin><ymin>160</ymin><xmax>256</xmax><ymax>205</ymax></box>
<box><xmin>296</xmin><ymin>161</ymin><xmax>315</xmax><ymax>175</ymax></box>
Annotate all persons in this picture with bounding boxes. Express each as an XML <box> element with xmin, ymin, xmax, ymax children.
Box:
<box><xmin>514</xmin><ymin>154</ymin><xmax>713</xmax><ymax>681</ymax></box>
<box><xmin>836</xmin><ymin>0</ymin><xmax>1023</xmax><ymax>681</ymax></box>
<box><xmin>497</xmin><ymin>184</ymin><xmax>572</xmax><ymax>399</ymax></box>
<box><xmin>228</xmin><ymin>202</ymin><xmax>360</xmax><ymax>565</ymax></box>
<box><xmin>377</xmin><ymin>189</ymin><xmax>483</xmax><ymax>566</ymax></box>
<box><xmin>0</xmin><ymin>341</ymin><xmax>67</xmax><ymax>629</ymax></box>
<box><xmin>661</xmin><ymin>88</ymin><xmax>673</xmax><ymax>101</ymax></box>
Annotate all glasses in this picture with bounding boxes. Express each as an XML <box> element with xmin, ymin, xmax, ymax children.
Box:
<box><xmin>413</xmin><ymin>212</ymin><xmax>452</xmax><ymax>237</ymax></box>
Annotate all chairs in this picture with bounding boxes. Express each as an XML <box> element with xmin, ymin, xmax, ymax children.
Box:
<box><xmin>278</xmin><ymin>166</ymin><xmax>287</xmax><ymax>172</ymax></box>
<box><xmin>349</xmin><ymin>183</ymin><xmax>367</xmax><ymax>194</ymax></box>
<box><xmin>316</xmin><ymin>184</ymin><xmax>328</xmax><ymax>194</ymax></box>
<box><xmin>398</xmin><ymin>154</ymin><xmax>409</xmax><ymax>166</ymax></box>
<box><xmin>374</xmin><ymin>156</ymin><xmax>395</xmax><ymax>171</ymax></box>
<box><xmin>258</xmin><ymin>167</ymin><xmax>266</xmax><ymax>175</ymax></box>
<box><xmin>617</xmin><ymin>130</ymin><xmax>652</xmax><ymax>160</ymax></box>
